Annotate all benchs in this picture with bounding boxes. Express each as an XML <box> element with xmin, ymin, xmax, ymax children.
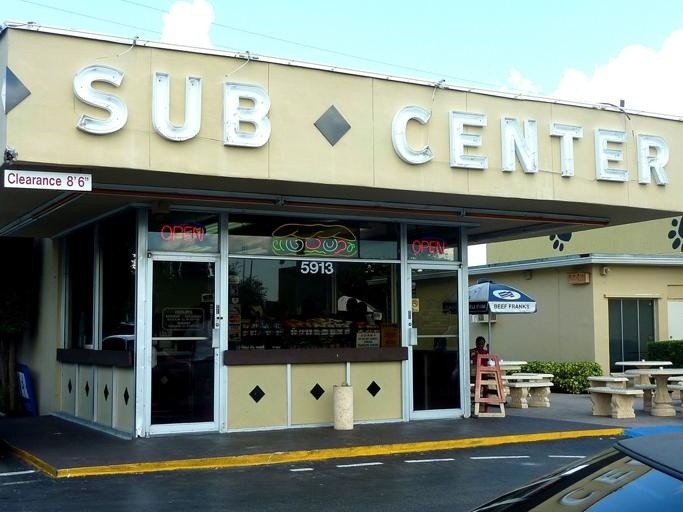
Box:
<box><xmin>586</xmin><ymin>373</ymin><xmax>683</xmax><ymax>419</ymax></box>
<box><xmin>500</xmin><ymin>373</ymin><xmax>555</xmax><ymax>408</ymax></box>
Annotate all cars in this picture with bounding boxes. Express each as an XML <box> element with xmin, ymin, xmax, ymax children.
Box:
<box><xmin>471</xmin><ymin>428</ymin><xmax>683</xmax><ymax>512</ymax></box>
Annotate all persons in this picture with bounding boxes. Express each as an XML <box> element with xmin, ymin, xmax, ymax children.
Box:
<box><xmin>428</xmin><ymin>336</ymin><xmax>455</xmax><ymax>380</ymax></box>
<box><xmin>335</xmin><ymin>297</ymin><xmax>359</xmax><ymax>319</ymax></box>
<box><xmin>469</xmin><ymin>334</ymin><xmax>491</xmax><ymax>365</ymax></box>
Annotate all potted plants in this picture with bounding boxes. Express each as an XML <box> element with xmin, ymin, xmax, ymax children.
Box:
<box><xmin>335</xmin><ymin>383</ymin><xmax>354</xmax><ymax>431</ymax></box>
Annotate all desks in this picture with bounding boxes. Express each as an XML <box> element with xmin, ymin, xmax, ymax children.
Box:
<box><xmin>499</xmin><ymin>362</ymin><xmax>529</xmax><ymax>396</ymax></box>
<box><xmin>615</xmin><ymin>361</ymin><xmax>674</xmax><ymax>397</ymax></box>
<box><xmin>625</xmin><ymin>369</ymin><xmax>683</xmax><ymax>417</ymax></box>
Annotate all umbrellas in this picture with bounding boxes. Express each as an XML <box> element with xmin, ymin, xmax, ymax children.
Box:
<box><xmin>440</xmin><ymin>281</ymin><xmax>537</xmax><ymax>356</ymax></box>
